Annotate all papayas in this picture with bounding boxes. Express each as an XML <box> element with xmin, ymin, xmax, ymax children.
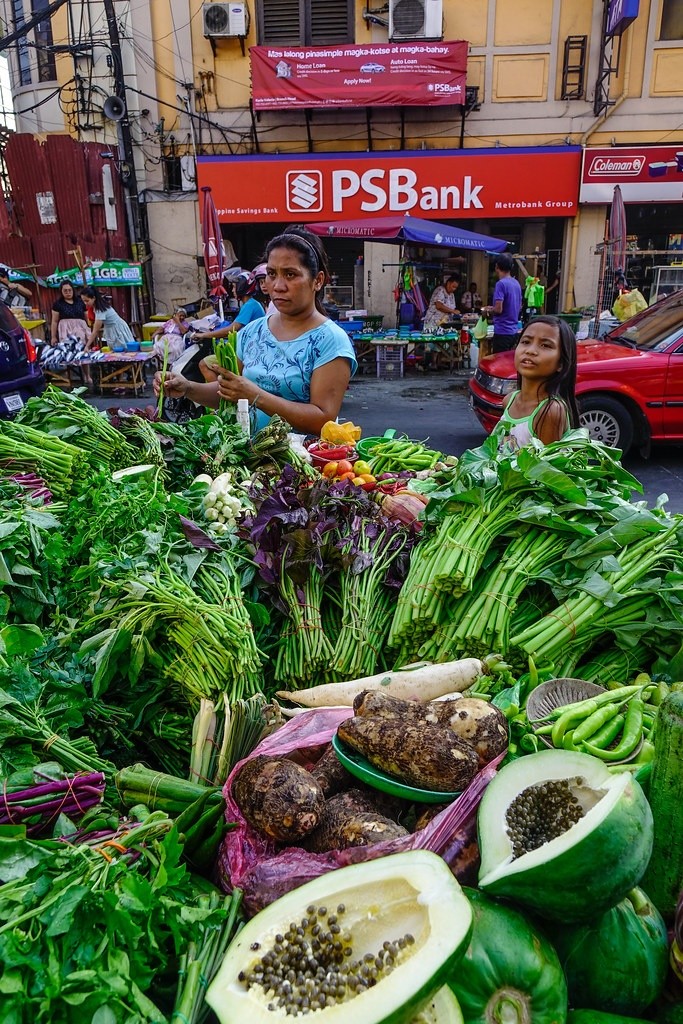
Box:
<box><xmin>410</xmin><ymin>883</ymin><xmax>683</xmax><ymax>1024</ymax></box>
<box><xmin>203</xmin><ymin>851</ymin><xmax>474</xmax><ymax>1024</ymax></box>
<box><xmin>476</xmin><ymin>748</ymin><xmax>653</xmax><ymax>926</ymax></box>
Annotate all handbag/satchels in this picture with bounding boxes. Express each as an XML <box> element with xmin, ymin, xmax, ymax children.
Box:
<box><xmin>474</xmin><ymin>315</ymin><xmax>487</xmax><ymax>339</ymax></box>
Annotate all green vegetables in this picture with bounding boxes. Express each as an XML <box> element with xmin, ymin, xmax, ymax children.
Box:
<box><xmin>0</xmin><ymin>465</ymin><xmax>422</xmax><ymax>1024</ymax></box>
<box><xmin>0</xmin><ymin>326</ymin><xmax>318</xmax><ymax>497</ymax></box>
<box><xmin>388</xmin><ymin>439</ymin><xmax>682</xmax><ymax>686</ymax></box>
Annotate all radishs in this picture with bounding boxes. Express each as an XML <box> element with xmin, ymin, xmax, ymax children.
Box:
<box><xmin>276</xmin><ymin>658</ymin><xmax>486</xmax><ymax>717</ymax></box>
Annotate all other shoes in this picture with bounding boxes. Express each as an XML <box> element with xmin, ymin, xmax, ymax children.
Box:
<box><xmin>431</xmin><ymin>368</ymin><xmax>440</xmax><ymax>372</ymax></box>
<box><xmin>112</xmin><ymin>386</ymin><xmax>125</xmax><ymax>392</ymax></box>
<box><xmin>437</xmin><ymin>366</ymin><xmax>449</xmax><ymax>370</ymax></box>
<box><xmin>85</xmin><ymin>376</ymin><xmax>93</xmax><ymax>383</ymax></box>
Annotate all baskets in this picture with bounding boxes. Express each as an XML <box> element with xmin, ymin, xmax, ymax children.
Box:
<box><xmin>526</xmin><ymin>678</ymin><xmax>644</xmax><ymax>766</ymax></box>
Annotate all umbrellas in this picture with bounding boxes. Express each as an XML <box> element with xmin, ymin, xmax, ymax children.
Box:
<box><xmin>200</xmin><ymin>187</ymin><xmax>229</xmax><ymax>322</ymax></box>
<box><xmin>610</xmin><ymin>185</ymin><xmax>627</xmax><ymax>296</ymax></box>
<box><xmin>304</xmin><ymin>211</ymin><xmax>515</xmax><ymax>328</ymax></box>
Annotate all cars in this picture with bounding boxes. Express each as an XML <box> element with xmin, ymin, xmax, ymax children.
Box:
<box><xmin>0</xmin><ymin>298</ymin><xmax>47</xmax><ymax>421</ymax></box>
<box><xmin>468</xmin><ymin>286</ymin><xmax>683</xmax><ymax>466</ymax></box>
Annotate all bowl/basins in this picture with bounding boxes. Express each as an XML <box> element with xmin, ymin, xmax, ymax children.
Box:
<box><xmin>140</xmin><ymin>341</ymin><xmax>153</xmax><ymax>352</ymax></box>
<box><xmin>339</xmin><ymin>320</ymin><xmax>365</xmax><ymax>331</ymax></box>
<box><xmin>126</xmin><ymin>341</ymin><xmax>139</xmax><ymax>351</ymax></box>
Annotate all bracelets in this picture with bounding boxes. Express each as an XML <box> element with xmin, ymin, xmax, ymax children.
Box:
<box><xmin>472</xmin><ymin>307</ymin><xmax>475</xmax><ymax>313</ymax></box>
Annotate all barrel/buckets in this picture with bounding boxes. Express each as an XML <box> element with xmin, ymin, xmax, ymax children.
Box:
<box><xmin>400</xmin><ymin>303</ymin><xmax>415</xmax><ymax>324</ymax></box>
<box><xmin>307</xmin><ymin>444</ymin><xmax>360</xmax><ymax>473</ymax></box>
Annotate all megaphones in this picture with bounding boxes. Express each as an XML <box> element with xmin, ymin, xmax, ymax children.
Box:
<box><xmin>104</xmin><ymin>96</ymin><xmax>126</xmax><ymax>120</ymax></box>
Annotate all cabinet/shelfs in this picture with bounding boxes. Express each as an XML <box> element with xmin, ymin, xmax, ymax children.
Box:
<box><xmin>648</xmin><ymin>266</ymin><xmax>683</xmax><ymax>307</ymax></box>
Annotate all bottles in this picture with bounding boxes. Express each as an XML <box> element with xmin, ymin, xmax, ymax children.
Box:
<box><xmin>470</xmin><ymin>343</ymin><xmax>480</xmax><ymax>368</ymax></box>
<box><xmin>461</xmin><ymin>325</ymin><xmax>469</xmax><ymax>345</ymax></box>
<box><xmin>236</xmin><ymin>399</ymin><xmax>250</xmax><ymax>440</ymax></box>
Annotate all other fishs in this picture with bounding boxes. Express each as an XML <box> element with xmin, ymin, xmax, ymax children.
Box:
<box><xmin>39</xmin><ymin>333</ymin><xmax>106</xmax><ymax>364</ymax></box>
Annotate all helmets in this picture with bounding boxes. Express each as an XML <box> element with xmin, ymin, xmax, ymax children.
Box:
<box><xmin>255</xmin><ymin>265</ymin><xmax>268</xmax><ymax>282</ymax></box>
<box><xmin>229</xmin><ymin>270</ymin><xmax>257</xmax><ymax>297</ymax></box>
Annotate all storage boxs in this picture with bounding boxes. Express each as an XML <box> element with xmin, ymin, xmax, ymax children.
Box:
<box><xmin>376</xmin><ymin>346</ymin><xmax>403</xmax><ymax>378</ymax></box>
<box><xmin>142</xmin><ymin>322</ymin><xmax>166</xmax><ymax>340</ymax></box>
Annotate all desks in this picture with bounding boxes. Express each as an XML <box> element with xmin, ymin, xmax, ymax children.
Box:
<box><xmin>350</xmin><ymin>330</ymin><xmax>472</xmax><ymax>375</ymax></box>
<box><xmin>40</xmin><ymin>350</ymin><xmax>157</xmax><ymax>397</ymax></box>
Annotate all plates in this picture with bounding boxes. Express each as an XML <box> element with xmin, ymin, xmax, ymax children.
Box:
<box><xmin>525</xmin><ymin>677</ymin><xmax>645</xmax><ymax>766</ymax></box>
<box><xmin>331</xmin><ymin>733</ymin><xmax>467</xmax><ymax>803</ymax></box>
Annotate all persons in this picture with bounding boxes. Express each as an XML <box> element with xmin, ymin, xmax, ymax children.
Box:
<box><xmin>422</xmin><ymin>274</ymin><xmax>460</xmax><ymax>371</ymax></box>
<box><xmin>0</xmin><ymin>267</ymin><xmax>33</xmax><ymax>300</ymax></box>
<box><xmin>491</xmin><ymin>314</ymin><xmax>581</xmax><ymax>459</ymax></box>
<box><xmin>461</xmin><ymin>282</ymin><xmax>483</xmax><ymax>314</ymax></box>
<box><xmin>152</xmin><ymin>227</ymin><xmax>358</xmax><ymax>440</ymax></box>
<box><xmin>51</xmin><ymin>279</ymin><xmax>147</xmax><ymax>391</ymax></box>
<box><xmin>480</xmin><ymin>252</ymin><xmax>522</xmax><ymax>354</ymax></box>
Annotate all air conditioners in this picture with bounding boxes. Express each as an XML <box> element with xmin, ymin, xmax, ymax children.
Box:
<box><xmin>202</xmin><ymin>2</ymin><xmax>246</xmax><ymax>39</ymax></box>
<box><xmin>388</xmin><ymin>0</ymin><xmax>443</xmax><ymax>43</ymax></box>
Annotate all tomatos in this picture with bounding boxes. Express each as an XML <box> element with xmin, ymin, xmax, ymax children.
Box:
<box><xmin>322</xmin><ymin>459</ymin><xmax>377</xmax><ymax>486</ymax></box>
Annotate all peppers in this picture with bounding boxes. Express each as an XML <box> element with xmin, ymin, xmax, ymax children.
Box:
<box><xmin>505</xmin><ymin>655</ymin><xmax>683</xmax><ymax>773</ymax></box>
<box><xmin>368</xmin><ymin>433</ymin><xmax>442</xmax><ymax>475</ymax></box>
